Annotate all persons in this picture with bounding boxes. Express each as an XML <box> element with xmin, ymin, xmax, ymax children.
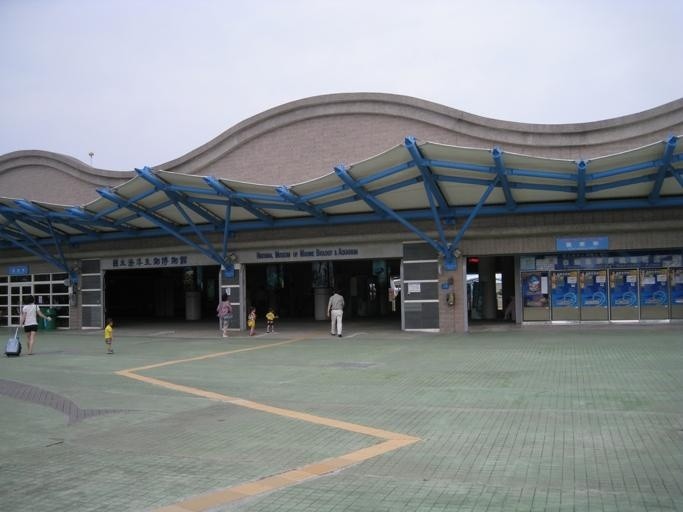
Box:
<box><xmin>245</xmin><ymin>305</ymin><xmax>256</xmax><ymax>335</ymax></box>
<box><xmin>265</xmin><ymin>307</ymin><xmax>279</xmax><ymax>332</ymax></box>
<box><xmin>327</xmin><ymin>288</ymin><xmax>344</xmax><ymax>337</ymax></box>
<box><xmin>17</xmin><ymin>294</ymin><xmax>52</xmax><ymax>355</ymax></box>
<box><xmin>103</xmin><ymin>318</ymin><xmax>114</xmax><ymax>354</ymax></box>
<box><xmin>215</xmin><ymin>292</ymin><xmax>231</xmax><ymax>340</ymax></box>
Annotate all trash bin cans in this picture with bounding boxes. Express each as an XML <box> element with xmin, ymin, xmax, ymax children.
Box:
<box><xmin>37</xmin><ymin>309</ymin><xmax>57</xmax><ymax>330</ymax></box>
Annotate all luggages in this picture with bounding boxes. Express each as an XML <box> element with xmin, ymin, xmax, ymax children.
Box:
<box><xmin>5</xmin><ymin>327</ymin><xmax>20</xmax><ymax>356</ymax></box>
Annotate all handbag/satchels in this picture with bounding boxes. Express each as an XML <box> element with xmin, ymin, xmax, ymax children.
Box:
<box><xmin>223</xmin><ymin>313</ymin><xmax>233</xmax><ymax>320</ymax></box>
